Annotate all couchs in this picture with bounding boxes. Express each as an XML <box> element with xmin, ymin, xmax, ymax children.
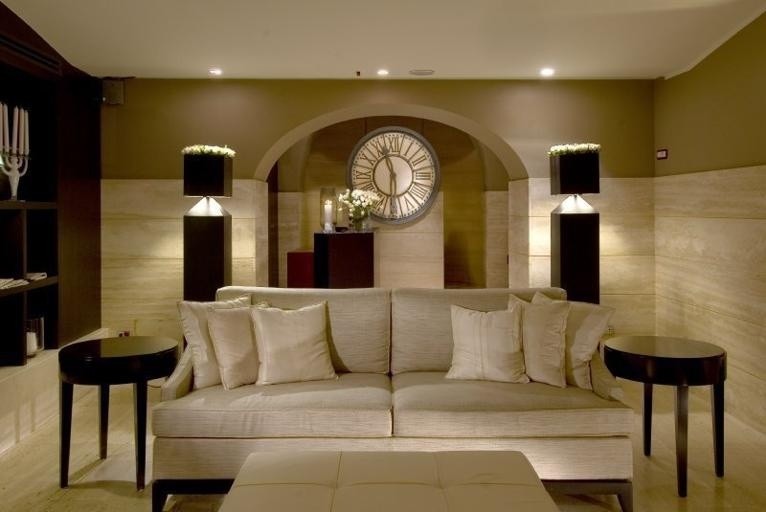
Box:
<box><xmin>152</xmin><ymin>286</ymin><xmax>643</xmax><ymax>512</ymax></box>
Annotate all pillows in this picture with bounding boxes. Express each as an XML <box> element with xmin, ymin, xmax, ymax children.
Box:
<box><xmin>445</xmin><ymin>291</ymin><xmax>615</xmax><ymax>391</ymax></box>
<box><xmin>216</xmin><ymin>286</ymin><xmax>390</xmax><ymax>375</ymax></box>
<box><xmin>175</xmin><ymin>295</ymin><xmax>339</xmax><ymax>389</ymax></box>
<box><xmin>391</xmin><ymin>288</ymin><xmax>567</xmax><ymax>374</ymax></box>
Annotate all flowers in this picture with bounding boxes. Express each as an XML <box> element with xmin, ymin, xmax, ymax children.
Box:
<box><xmin>336</xmin><ymin>188</ymin><xmax>380</xmax><ymax>223</ymax></box>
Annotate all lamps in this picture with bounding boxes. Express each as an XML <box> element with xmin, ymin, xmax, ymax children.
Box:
<box><xmin>182</xmin><ymin>145</ymin><xmax>236</xmax><ymax>302</ymax></box>
<box><xmin>548</xmin><ymin>144</ymin><xmax>599</xmax><ymax>305</ymax></box>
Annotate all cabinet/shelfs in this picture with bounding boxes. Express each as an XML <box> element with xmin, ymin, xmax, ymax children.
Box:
<box><xmin>287</xmin><ymin>251</ymin><xmax>315</xmax><ymax>288</ymax></box>
<box><xmin>0</xmin><ymin>1</ymin><xmax>59</xmax><ymax>368</ymax></box>
<box><xmin>314</xmin><ymin>232</ymin><xmax>374</xmax><ymax>288</ymax></box>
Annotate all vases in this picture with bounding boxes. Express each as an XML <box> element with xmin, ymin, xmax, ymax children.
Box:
<box><xmin>352</xmin><ymin>219</ymin><xmax>364</xmax><ymax>232</ymax></box>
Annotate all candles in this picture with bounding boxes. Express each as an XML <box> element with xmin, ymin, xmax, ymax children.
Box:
<box><xmin>324</xmin><ymin>200</ymin><xmax>333</xmax><ymax>231</ymax></box>
<box><xmin>27</xmin><ymin>332</ymin><xmax>38</xmax><ymax>355</ymax></box>
<box><xmin>0</xmin><ymin>103</ymin><xmax>31</xmax><ymax>155</ymax></box>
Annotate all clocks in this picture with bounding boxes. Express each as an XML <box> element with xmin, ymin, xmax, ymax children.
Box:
<box><xmin>345</xmin><ymin>125</ymin><xmax>440</xmax><ymax>226</ymax></box>
